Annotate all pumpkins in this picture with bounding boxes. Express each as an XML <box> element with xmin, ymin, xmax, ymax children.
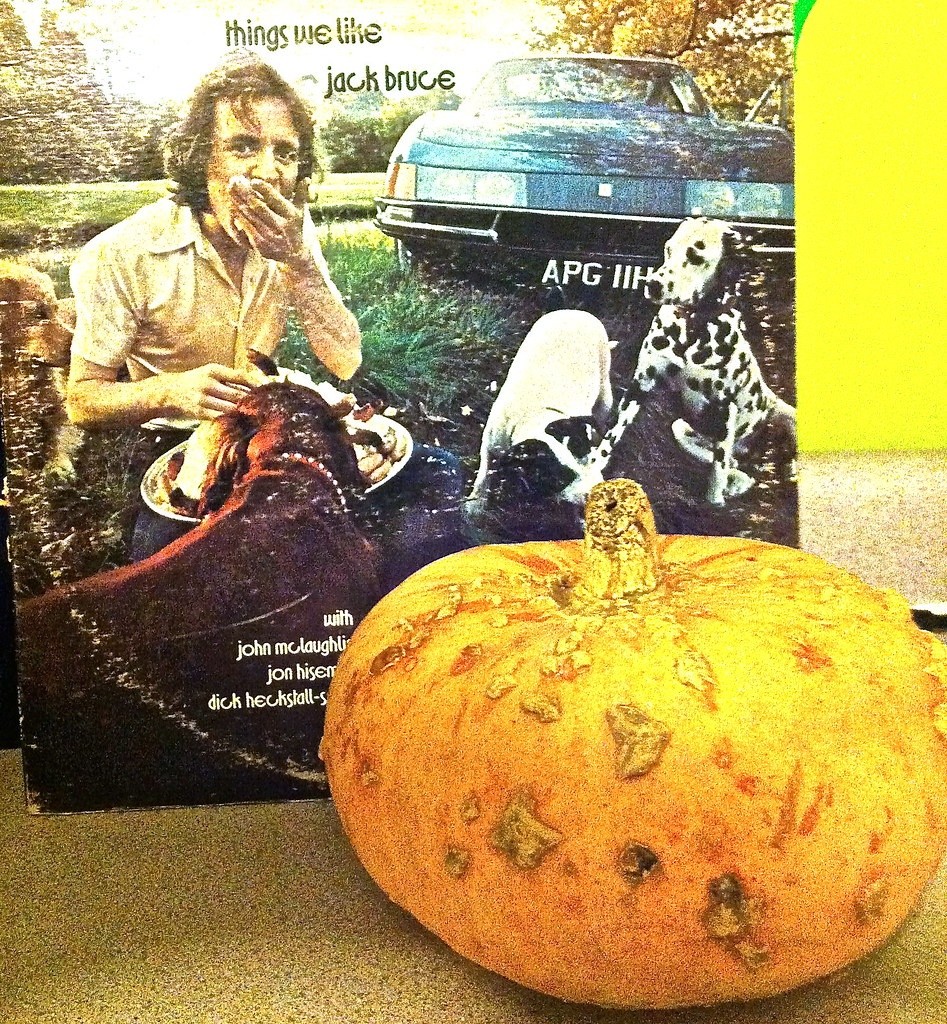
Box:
<box><xmin>318</xmin><ymin>476</ymin><xmax>947</xmax><ymax>1012</ymax></box>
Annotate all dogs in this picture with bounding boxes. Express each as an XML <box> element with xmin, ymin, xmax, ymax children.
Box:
<box><xmin>17</xmin><ymin>214</ymin><xmax>801</xmax><ymax>814</ymax></box>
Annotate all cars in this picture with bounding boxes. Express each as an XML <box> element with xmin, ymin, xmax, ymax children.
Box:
<box><xmin>375</xmin><ymin>53</ymin><xmax>795</xmax><ymax>280</ymax></box>
